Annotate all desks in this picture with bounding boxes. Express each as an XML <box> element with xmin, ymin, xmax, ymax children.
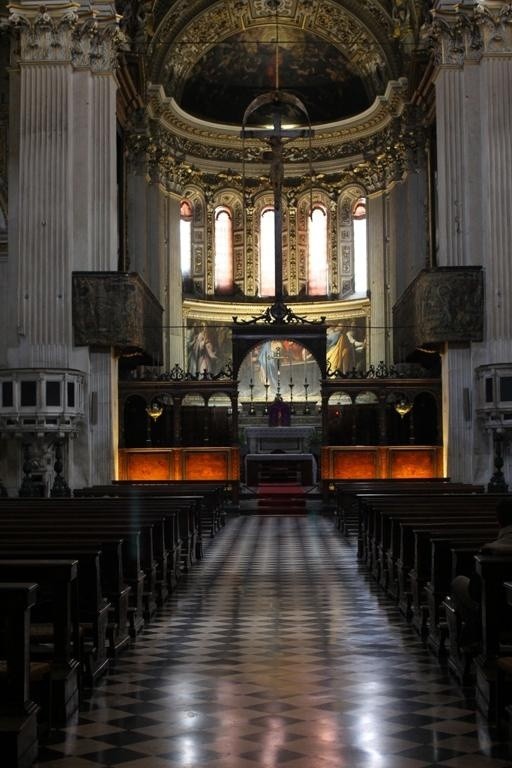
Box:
<box><xmin>244</xmin><ymin>454</ymin><xmax>317</xmax><ymax>485</ymax></box>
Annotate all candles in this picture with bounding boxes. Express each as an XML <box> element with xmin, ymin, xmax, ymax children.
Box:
<box><xmin>263</xmin><ymin>350</ymin><xmax>270</xmax><ymax>416</ymax></box>
<box><xmin>303</xmin><ymin>349</ymin><xmax>311</xmax><ymax>414</ymax></box>
<box><xmin>248</xmin><ymin>352</ymin><xmax>256</xmax><ymax>415</ymax></box>
<box><xmin>276</xmin><ymin>370</ymin><xmax>281</xmax><ymax>401</ymax></box>
<box><xmin>289</xmin><ymin>349</ymin><xmax>296</xmax><ymax>414</ymax></box>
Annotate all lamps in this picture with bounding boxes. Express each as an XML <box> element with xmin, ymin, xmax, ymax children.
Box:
<box><xmin>146</xmin><ymin>404</ymin><xmax>163</xmax><ymax>422</ymax></box>
<box><xmin>393</xmin><ymin>401</ymin><xmax>414</xmax><ymax>418</ymax></box>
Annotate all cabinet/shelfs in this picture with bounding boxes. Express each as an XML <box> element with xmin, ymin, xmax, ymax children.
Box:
<box><xmin>321</xmin><ymin>379</ymin><xmax>443</xmax><ymax>479</ymax></box>
<box><xmin>119</xmin><ymin>380</ymin><xmax>241</xmax><ymax>479</ymax></box>
